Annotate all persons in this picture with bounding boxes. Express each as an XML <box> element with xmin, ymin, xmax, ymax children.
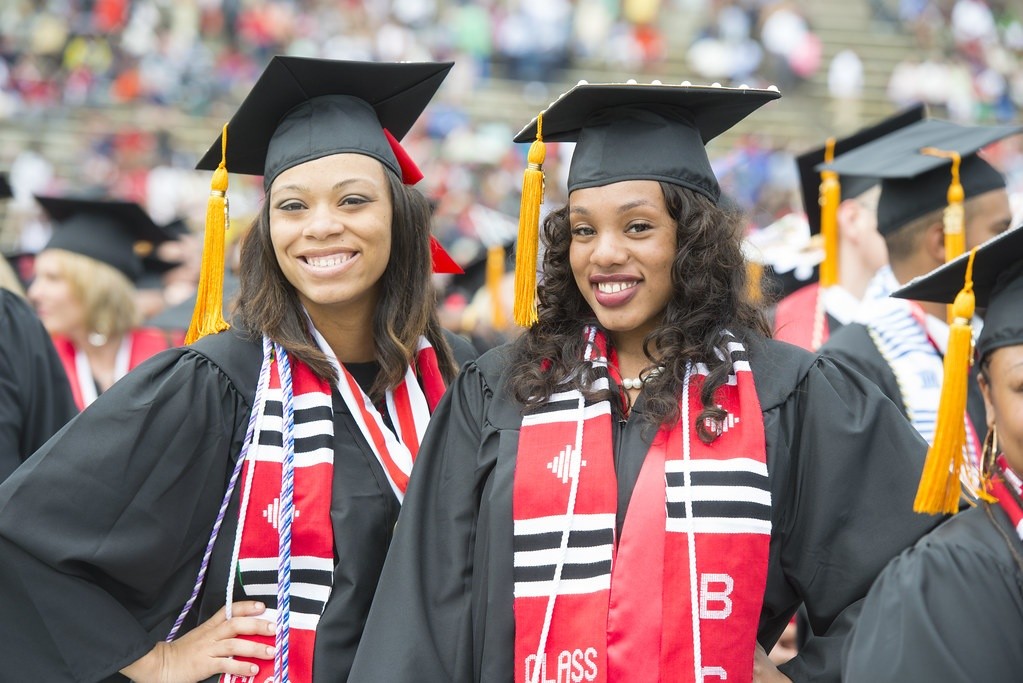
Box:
<box><xmin>345</xmin><ymin>80</ymin><xmax>969</xmax><ymax>683</ymax></box>
<box><xmin>0</xmin><ymin>56</ymin><xmax>480</xmax><ymax>683</ymax></box>
<box><xmin>0</xmin><ymin>0</ymin><xmax>1023</xmax><ymax>683</ymax></box>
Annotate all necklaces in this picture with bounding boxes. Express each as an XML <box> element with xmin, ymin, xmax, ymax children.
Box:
<box><xmin>618</xmin><ymin>366</ymin><xmax>665</xmax><ymax>389</ymax></box>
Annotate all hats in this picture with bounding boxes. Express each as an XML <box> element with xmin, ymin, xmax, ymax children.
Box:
<box><xmin>184</xmin><ymin>55</ymin><xmax>454</xmax><ymax>346</ymax></box>
<box><xmin>796</xmin><ymin>103</ymin><xmax>928</xmax><ymax>288</ymax></box>
<box><xmin>513</xmin><ymin>79</ymin><xmax>782</xmax><ymax>328</ymax></box>
<box><xmin>889</xmin><ymin>226</ymin><xmax>1023</xmax><ymax>515</ymax></box>
<box><xmin>34</xmin><ymin>195</ymin><xmax>165</xmax><ymax>291</ymax></box>
<box><xmin>814</xmin><ymin>118</ymin><xmax>1023</xmax><ymax>323</ymax></box>
